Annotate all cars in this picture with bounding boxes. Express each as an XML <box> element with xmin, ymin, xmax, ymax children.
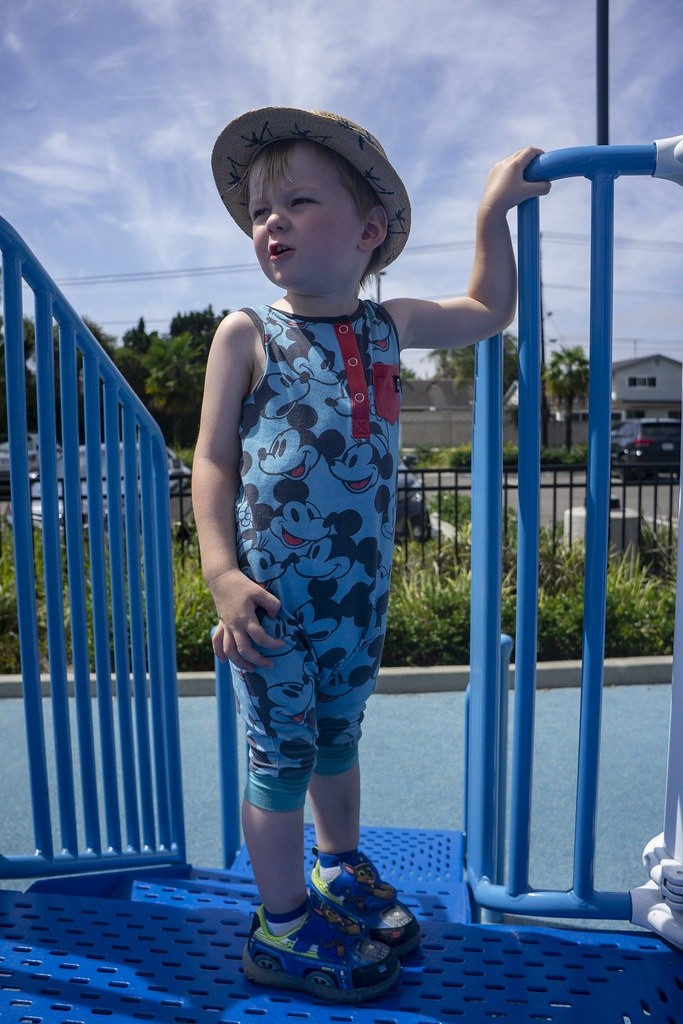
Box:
<box><xmin>0</xmin><ymin>432</ymin><xmax>191</xmax><ymax>530</ymax></box>
<box><xmin>392</xmin><ymin>454</ymin><xmax>432</xmax><ymax>545</ymax></box>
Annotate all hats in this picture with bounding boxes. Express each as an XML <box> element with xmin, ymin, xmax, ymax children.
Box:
<box><xmin>211</xmin><ymin>105</ymin><xmax>412</xmax><ymax>276</ymax></box>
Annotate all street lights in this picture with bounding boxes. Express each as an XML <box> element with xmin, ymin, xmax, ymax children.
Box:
<box><xmin>371</xmin><ymin>269</ymin><xmax>387</xmax><ymax>303</ymax></box>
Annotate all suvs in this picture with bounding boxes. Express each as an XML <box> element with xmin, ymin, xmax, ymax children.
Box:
<box><xmin>608</xmin><ymin>415</ymin><xmax>680</xmax><ymax>481</ymax></box>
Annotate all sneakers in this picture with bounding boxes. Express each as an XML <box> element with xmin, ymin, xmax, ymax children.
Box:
<box><xmin>242</xmin><ymin>897</ymin><xmax>400</xmax><ymax>1002</ymax></box>
<box><xmin>308</xmin><ymin>845</ymin><xmax>419</xmax><ymax>946</ymax></box>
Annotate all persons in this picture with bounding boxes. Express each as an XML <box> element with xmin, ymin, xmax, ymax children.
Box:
<box><xmin>190</xmin><ymin>106</ymin><xmax>550</xmax><ymax>1002</ymax></box>
